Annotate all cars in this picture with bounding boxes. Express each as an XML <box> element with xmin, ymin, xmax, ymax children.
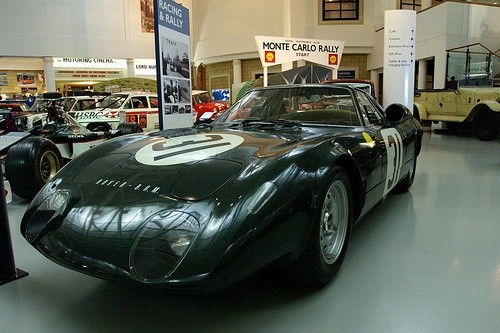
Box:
<box><xmin>0</xmin><ymin>85</ymin><xmax>233</xmax><ymax>199</ymax></box>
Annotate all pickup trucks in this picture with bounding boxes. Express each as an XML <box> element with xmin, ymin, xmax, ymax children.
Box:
<box><xmin>414</xmin><ymin>41</ymin><xmax>500</xmax><ymax>141</ymax></box>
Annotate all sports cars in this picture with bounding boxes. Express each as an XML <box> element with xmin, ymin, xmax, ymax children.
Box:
<box><xmin>20</xmin><ymin>77</ymin><xmax>426</xmax><ymax>295</ymax></box>
